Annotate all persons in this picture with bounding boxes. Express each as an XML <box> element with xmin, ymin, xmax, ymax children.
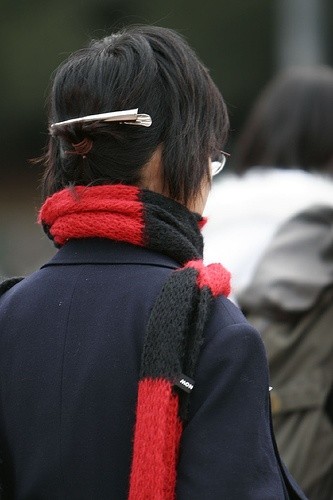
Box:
<box><xmin>0</xmin><ymin>24</ymin><xmax>307</xmax><ymax>500</ymax></box>
<box><xmin>202</xmin><ymin>63</ymin><xmax>333</xmax><ymax>500</ymax></box>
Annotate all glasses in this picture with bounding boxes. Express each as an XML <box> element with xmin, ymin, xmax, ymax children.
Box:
<box><xmin>210</xmin><ymin>149</ymin><xmax>231</xmax><ymax>178</ymax></box>
